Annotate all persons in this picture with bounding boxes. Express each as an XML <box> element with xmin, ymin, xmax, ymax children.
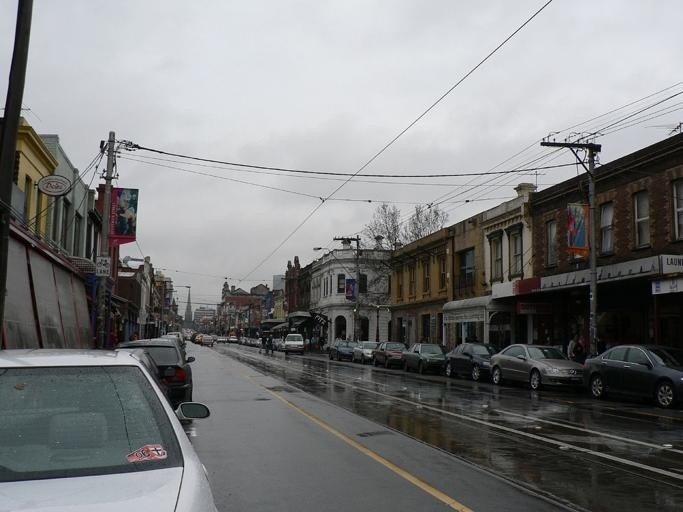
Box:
<box><xmin>264</xmin><ymin>334</ymin><xmax>274</xmax><ymax>354</ymax></box>
<box><xmin>258</xmin><ymin>333</ymin><xmax>268</xmax><ymax>353</ymax></box>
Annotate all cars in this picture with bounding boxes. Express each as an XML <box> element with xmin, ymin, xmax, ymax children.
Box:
<box><xmin>225</xmin><ymin>333</ymin><xmax>284</xmax><ymax>356</ymax></box>
<box><xmin>216</xmin><ymin>336</ymin><xmax>226</xmax><ymax>344</ymax></box>
<box><xmin>0</xmin><ymin>332</ymin><xmax>217</xmax><ymax>512</ymax></box>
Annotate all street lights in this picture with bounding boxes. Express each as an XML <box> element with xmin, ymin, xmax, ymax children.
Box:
<box><xmin>313</xmin><ymin>235</ymin><xmax>361</xmax><ymax>340</ymax></box>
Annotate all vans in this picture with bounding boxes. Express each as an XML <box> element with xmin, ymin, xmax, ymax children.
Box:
<box><xmin>284</xmin><ymin>334</ymin><xmax>304</xmax><ymax>356</ymax></box>
